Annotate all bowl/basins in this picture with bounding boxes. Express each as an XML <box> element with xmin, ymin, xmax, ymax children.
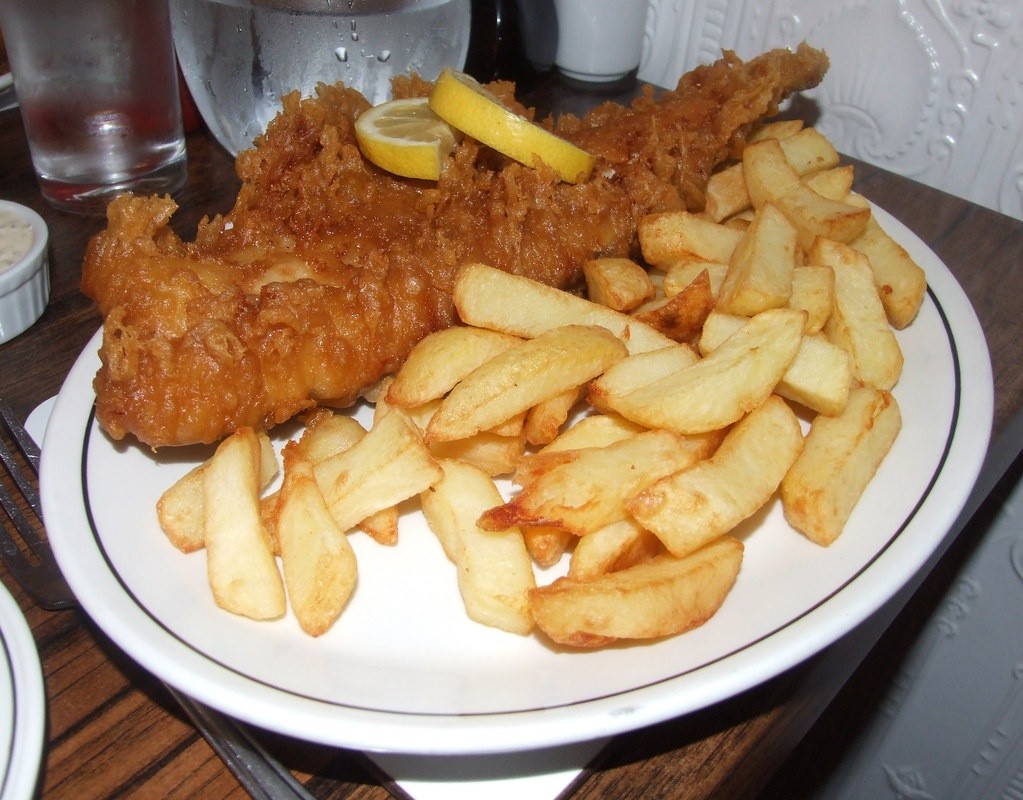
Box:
<box><xmin>170</xmin><ymin>1</ymin><xmax>471</xmax><ymax>158</ymax></box>
<box><xmin>0</xmin><ymin>198</ymin><xmax>52</xmax><ymax>346</ymax></box>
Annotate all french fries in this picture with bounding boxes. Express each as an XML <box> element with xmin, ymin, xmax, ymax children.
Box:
<box><xmin>156</xmin><ymin>120</ymin><xmax>928</xmax><ymax>651</ymax></box>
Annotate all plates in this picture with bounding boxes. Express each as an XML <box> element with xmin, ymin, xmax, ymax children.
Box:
<box><xmin>37</xmin><ymin>190</ymin><xmax>995</xmax><ymax>757</ymax></box>
<box><xmin>0</xmin><ymin>584</ymin><xmax>45</xmax><ymax>799</ymax></box>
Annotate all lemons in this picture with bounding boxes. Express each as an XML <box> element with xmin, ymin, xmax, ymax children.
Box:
<box><xmin>354</xmin><ymin>96</ymin><xmax>464</xmax><ymax>182</ymax></box>
<box><xmin>426</xmin><ymin>66</ymin><xmax>594</xmax><ymax>183</ymax></box>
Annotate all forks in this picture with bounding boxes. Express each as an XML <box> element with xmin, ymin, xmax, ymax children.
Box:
<box><xmin>1</xmin><ymin>402</ymin><xmax>315</xmax><ymax>800</ymax></box>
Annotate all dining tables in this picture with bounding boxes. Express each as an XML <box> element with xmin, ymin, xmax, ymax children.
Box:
<box><xmin>0</xmin><ymin>50</ymin><xmax>1023</xmax><ymax>800</ymax></box>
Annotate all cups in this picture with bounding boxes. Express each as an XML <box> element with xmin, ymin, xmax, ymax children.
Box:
<box><xmin>2</xmin><ymin>3</ymin><xmax>189</xmax><ymax>217</ymax></box>
<box><xmin>556</xmin><ymin>1</ymin><xmax>647</xmax><ymax>84</ymax></box>
